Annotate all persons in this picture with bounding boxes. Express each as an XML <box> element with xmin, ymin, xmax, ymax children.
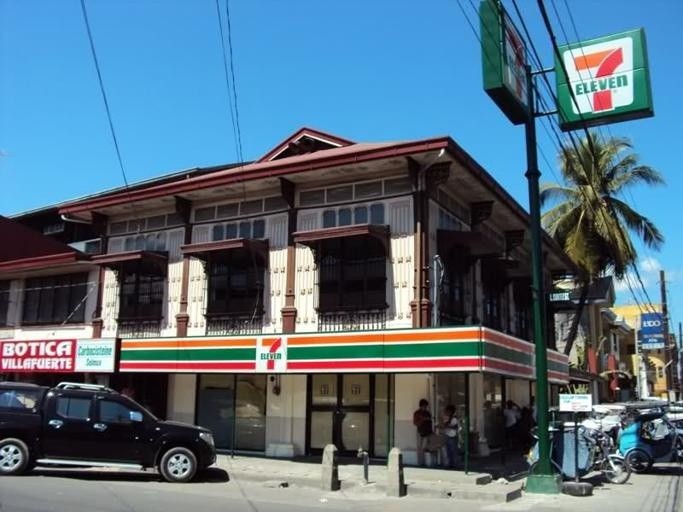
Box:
<box><xmin>481</xmin><ymin>393</ymin><xmax>538</xmax><ymax>456</ymax></box>
<box><xmin>437</xmin><ymin>404</ymin><xmax>461</xmax><ymax>468</ymax></box>
<box><xmin>412</xmin><ymin>399</ymin><xmax>439</xmax><ymax>468</ymax></box>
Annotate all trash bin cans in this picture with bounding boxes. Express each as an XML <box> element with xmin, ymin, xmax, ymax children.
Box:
<box><xmin>619</xmin><ymin>414</ymin><xmax>655</xmax><ymax>461</ymax></box>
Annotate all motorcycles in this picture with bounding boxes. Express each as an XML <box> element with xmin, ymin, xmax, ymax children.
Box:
<box><xmin>523</xmin><ymin>397</ymin><xmax>683</xmax><ymax>484</ymax></box>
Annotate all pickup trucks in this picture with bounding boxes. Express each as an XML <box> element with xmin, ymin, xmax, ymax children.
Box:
<box><xmin>0</xmin><ymin>380</ymin><xmax>218</xmax><ymax>483</ymax></box>
